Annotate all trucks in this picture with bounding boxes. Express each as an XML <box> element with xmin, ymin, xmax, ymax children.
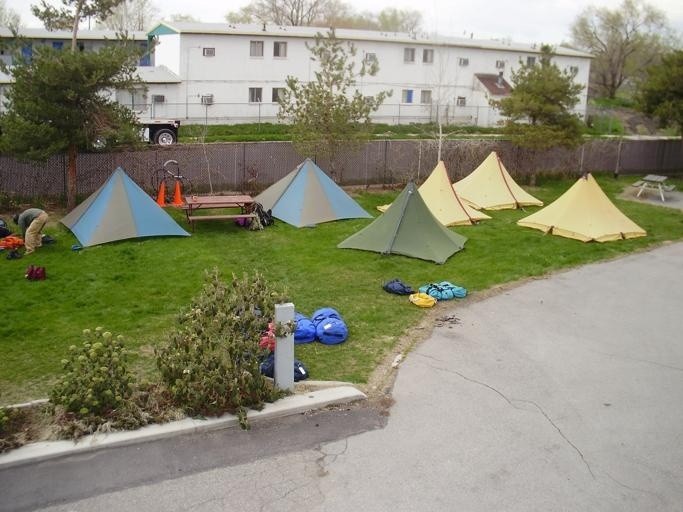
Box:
<box><xmin>89</xmin><ymin>109</ymin><xmax>180</xmax><ymax>153</ymax></box>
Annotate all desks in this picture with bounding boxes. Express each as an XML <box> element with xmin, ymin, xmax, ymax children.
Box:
<box><xmin>637</xmin><ymin>175</ymin><xmax>668</xmax><ymax>202</ymax></box>
<box><xmin>185</xmin><ymin>195</ymin><xmax>254</xmax><ymax>224</ymax></box>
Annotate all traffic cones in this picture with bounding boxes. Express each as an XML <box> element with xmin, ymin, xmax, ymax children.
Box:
<box><xmin>156</xmin><ymin>181</ymin><xmax>168</xmax><ymax>207</ymax></box>
<box><xmin>170</xmin><ymin>181</ymin><xmax>184</xmax><ymax>206</ymax></box>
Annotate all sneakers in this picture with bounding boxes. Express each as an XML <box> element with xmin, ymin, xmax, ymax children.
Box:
<box><xmin>6</xmin><ymin>250</ymin><xmax>22</xmax><ymax>260</ymax></box>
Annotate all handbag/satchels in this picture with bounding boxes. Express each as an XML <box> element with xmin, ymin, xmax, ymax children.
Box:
<box><xmin>23</xmin><ymin>264</ymin><xmax>47</xmax><ymax>282</ymax></box>
<box><xmin>235</xmin><ymin>201</ymin><xmax>275</xmax><ymax>233</ymax></box>
<box><xmin>260</xmin><ymin>350</ymin><xmax>309</xmax><ymax>383</ymax></box>
<box><xmin>0</xmin><ymin>218</ymin><xmax>11</xmax><ymax>238</ymax></box>
<box><xmin>382</xmin><ymin>278</ymin><xmax>412</xmax><ymax>296</ymax></box>
<box><xmin>408</xmin><ymin>280</ymin><xmax>467</xmax><ymax>309</ymax></box>
<box><xmin>294</xmin><ymin>307</ymin><xmax>349</xmax><ymax>347</ymax></box>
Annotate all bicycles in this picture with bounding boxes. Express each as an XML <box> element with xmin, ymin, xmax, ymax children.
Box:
<box><xmin>152</xmin><ymin>160</ymin><xmax>193</xmax><ymax>194</ymax></box>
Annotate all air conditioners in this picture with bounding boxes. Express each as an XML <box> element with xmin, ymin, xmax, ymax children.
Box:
<box><xmin>497</xmin><ymin>61</ymin><xmax>505</xmax><ymax>68</ymax></box>
<box><xmin>570</xmin><ymin>67</ymin><xmax>578</xmax><ymax>72</ymax></box>
<box><xmin>202</xmin><ymin>95</ymin><xmax>213</xmax><ymax>105</ymax></box>
<box><xmin>366</xmin><ymin>53</ymin><xmax>375</xmax><ymax>61</ymax></box>
<box><xmin>203</xmin><ymin>48</ymin><xmax>215</xmax><ymax>56</ymax></box>
<box><xmin>460</xmin><ymin>58</ymin><xmax>468</xmax><ymax>65</ymax></box>
<box><xmin>457</xmin><ymin>98</ymin><xmax>465</xmax><ymax>106</ymax></box>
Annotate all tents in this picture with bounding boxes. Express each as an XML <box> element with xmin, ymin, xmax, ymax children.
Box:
<box><xmin>56</xmin><ymin>164</ymin><xmax>195</xmax><ymax>247</ymax></box>
<box><xmin>451</xmin><ymin>149</ymin><xmax>545</xmax><ymax>214</ymax></box>
<box><xmin>249</xmin><ymin>155</ymin><xmax>375</xmax><ymax>231</ymax></box>
<box><xmin>513</xmin><ymin>167</ymin><xmax>648</xmax><ymax>245</ymax></box>
<box><xmin>375</xmin><ymin>156</ymin><xmax>494</xmax><ymax>229</ymax></box>
<box><xmin>334</xmin><ymin>175</ymin><xmax>471</xmax><ymax>267</ymax></box>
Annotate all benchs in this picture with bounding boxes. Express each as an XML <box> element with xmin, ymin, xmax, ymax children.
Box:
<box><xmin>188</xmin><ymin>215</ymin><xmax>257</xmax><ymax>233</ymax></box>
<box><xmin>182</xmin><ymin>203</ymin><xmax>240</xmax><ymax>218</ymax></box>
<box><xmin>664</xmin><ymin>184</ymin><xmax>676</xmax><ymax>192</ymax></box>
<box><xmin>632</xmin><ymin>180</ymin><xmax>645</xmax><ymax>187</ymax></box>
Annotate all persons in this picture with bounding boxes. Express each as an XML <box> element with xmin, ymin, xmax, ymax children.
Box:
<box><xmin>11</xmin><ymin>207</ymin><xmax>49</xmax><ymax>258</ymax></box>
